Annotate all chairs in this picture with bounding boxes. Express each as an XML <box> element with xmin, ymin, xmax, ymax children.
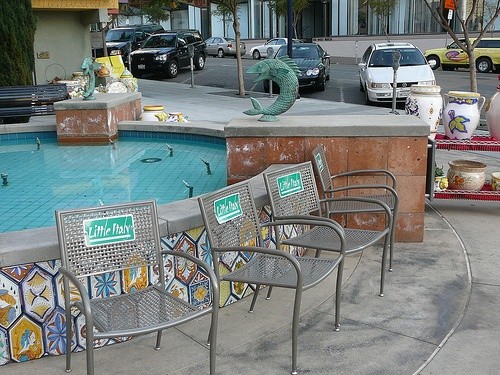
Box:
<box><xmin>55</xmin><ymin>200</ymin><xmax>221</xmax><ymax>375</ymax></box>
<box><xmin>311</xmin><ymin>144</ymin><xmax>399</xmax><ymax>272</ymax></box>
<box><xmin>262</xmin><ymin>159</ymin><xmax>393</xmax><ymax>327</ymax></box>
<box><xmin>196</xmin><ymin>180</ymin><xmax>347</xmax><ymax>375</ymax></box>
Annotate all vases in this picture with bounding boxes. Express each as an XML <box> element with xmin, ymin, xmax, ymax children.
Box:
<box><xmin>405</xmin><ymin>85</ymin><xmax>500</xmax><ymax>191</ymax></box>
<box><xmin>57</xmin><ymin>63</ymin><xmax>190</xmax><ymax>122</ymax></box>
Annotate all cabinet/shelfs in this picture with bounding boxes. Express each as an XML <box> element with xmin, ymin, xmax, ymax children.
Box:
<box><xmin>426</xmin><ymin>134</ymin><xmax>500</xmax><ymax>202</ymax></box>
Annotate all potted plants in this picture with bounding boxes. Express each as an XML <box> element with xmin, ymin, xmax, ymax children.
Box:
<box><xmin>434</xmin><ymin>161</ymin><xmax>448</xmax><ymax>192</ymax></box>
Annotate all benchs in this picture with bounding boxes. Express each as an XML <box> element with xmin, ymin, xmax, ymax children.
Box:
<box><xmin>0</xmin><ymin>82</ymin><xmax>71</xmax><ymax>117</ymax></box>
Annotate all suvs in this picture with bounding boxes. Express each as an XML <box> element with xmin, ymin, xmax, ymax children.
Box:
<box><xmin>129</xmin><ymin>29</ymin><xmax>206</xmax><ymax>78</ymax></box>
<box><xmin>93</xmin><ymin>23</ymin><xmax>165</xmax><ymax>69</ymax></box>
<box><xmin>422</xmin><ymin>37</ymin><xmax>500</xmax><ymax>73</ymax></box>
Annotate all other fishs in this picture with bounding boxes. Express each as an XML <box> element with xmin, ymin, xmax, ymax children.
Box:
<box><xmin>82</xmin><ymin>57</ymin><xmax>101</xmax><ymax>97</ymax></box>
<box><xmin>242</xmin><ymin>55</ymin><xmax>303</xmax><ymax>122</ymax></box>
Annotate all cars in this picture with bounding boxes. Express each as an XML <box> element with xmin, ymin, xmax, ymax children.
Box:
<box><xmin>359</xmin><ymin>43</ymin><xmax>437</xmax><ymax>105</ymax></box>
<box><xmin>205</xmin><ymin>37</ymin><xmax>247</xmax><ymax>58</ymax></box>
<box><xmin>263</xmin><ymin>42</ymin><xmax>330</xmax><ymax>94</ymax></box>
<box><xmin>249</xmin><ymin>38</ymin><xmax>305</xmax><ymax>59</ymax></box>
